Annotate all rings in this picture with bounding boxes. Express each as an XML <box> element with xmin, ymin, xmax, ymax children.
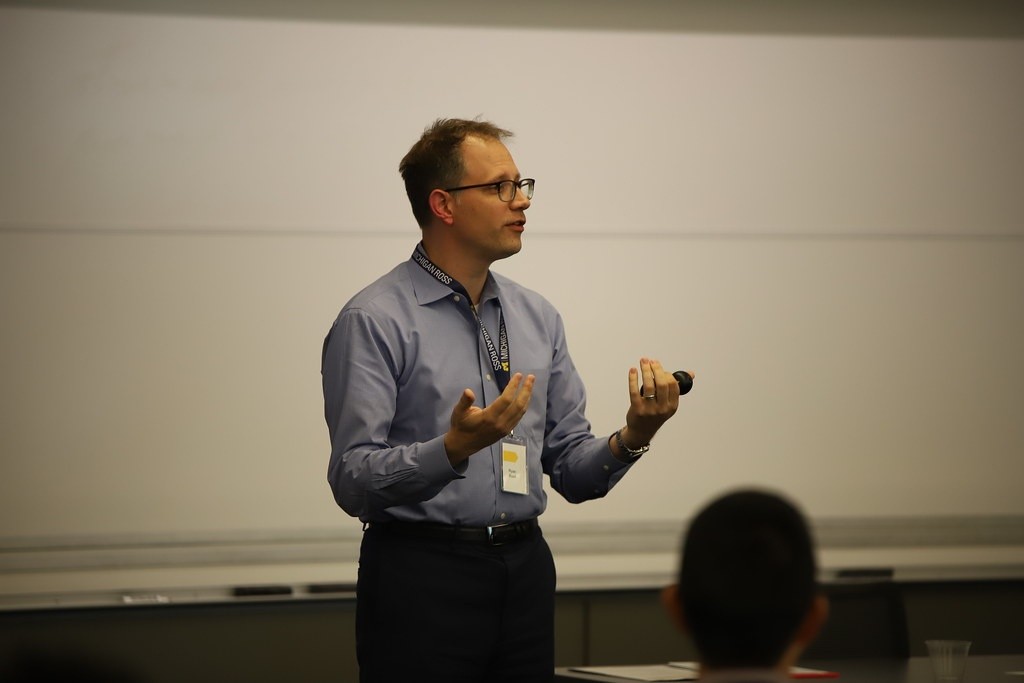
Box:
<box><xmin>643</xmin><ymin>395</ymin><xmax>655</xmax><ymax>400</ymax></box>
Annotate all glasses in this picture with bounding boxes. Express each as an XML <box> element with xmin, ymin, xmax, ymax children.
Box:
<box><xmin>443</xmin><ymin>178</ymin><xmax>536</xmax><ymax>202</ymax></box>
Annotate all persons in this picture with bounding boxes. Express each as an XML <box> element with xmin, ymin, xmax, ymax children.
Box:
<box><xmin>667</xmin><ymin>488</ymin><xmax>825</xmax><ymax>683</ymax></box>
<box><xmin>320</xmin><ymin>119</ymin><xmax>695</xmax><ymax>683</ymax></box>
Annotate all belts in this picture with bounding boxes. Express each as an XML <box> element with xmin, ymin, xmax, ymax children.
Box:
<box><xmin>368</xmin><ymin>519</ymin><xmax>539</xmax><ymax>547</ymax></box>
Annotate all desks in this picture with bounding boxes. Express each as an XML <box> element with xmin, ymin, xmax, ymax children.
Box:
<box><xmin>550</xmin><ymin>650</ymin><xmax>1024</xmax><ymax>681</ymax></box>
